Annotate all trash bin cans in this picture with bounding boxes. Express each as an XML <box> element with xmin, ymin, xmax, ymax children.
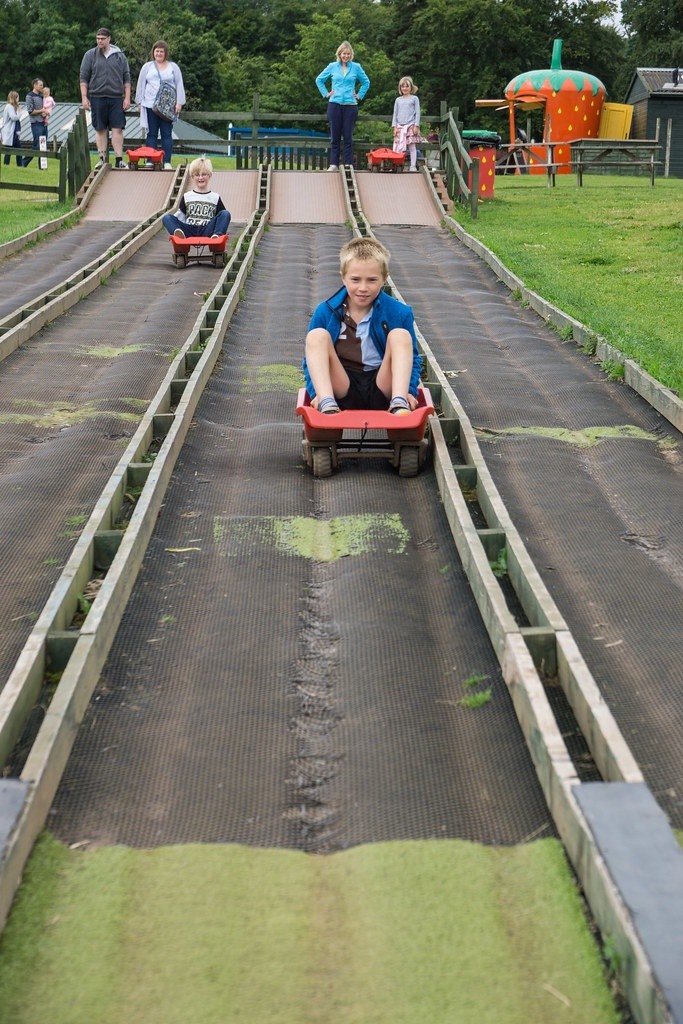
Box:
<box><xmin>461</xmin><ymin>130</ymin><xmax>501</xmax><ymax>200</ymax></box>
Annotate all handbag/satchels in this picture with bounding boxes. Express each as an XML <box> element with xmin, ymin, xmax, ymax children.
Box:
<box><xmin>152</xmin><ymin>82</ymin><xmax>179</xmax><ymax>123</ymax></box>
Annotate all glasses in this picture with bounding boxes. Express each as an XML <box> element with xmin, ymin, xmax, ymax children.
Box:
<box><xmin>96</xmin><ymin>36</ymin><xmax>109</xmax><ymax>39</ymax></box>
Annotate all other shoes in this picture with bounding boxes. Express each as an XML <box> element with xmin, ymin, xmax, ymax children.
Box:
<box><xmin>211</xmin><ymin>235</ymin><xmax>219</xmax><ymax>238</ymax></box>
<box><xmin>317</xmin><ymin>397</ymin><xmax>341</xmax><ymax>415</ymax></box>
<box><xmin>115</xmin><ymin>161</ymin><xmax>129</xmax><ymax>169</ymax></box>
<box><xmin>327</xmin><ymin>164</ymin><xmax>338</xmax><ymax>172</ymax></box>
<box><xmin>174</xmin><ymin>229</ymin><xmax>185</xmax><ymax>238</ymax></box>
<box><xmin>163</xmin><ymin>162</ymin><xmax>172</xmax><ymax>169</ymax></box>
<box><xmin>144</xmin><ymin>162</ymin><xmax>154</xmax><ymax>168</ymax></box>
<box><xmin>409</xmin><ymin>166</ymin><xmax>417</xmax><ymax>171</ymax></box>
<box><xmin>388</xmin><ymin>396</ymin><xmax>410</xmax><ymax>412</ymax></box>
<box><xmin>95</xmin><ymin>159</ymin><xmax>104</xmax><ymax>170</ymax></box>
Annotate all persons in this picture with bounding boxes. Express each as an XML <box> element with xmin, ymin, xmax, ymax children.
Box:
<box><xmin>391</xmin><ymin>76</ymin><xmax>429</xmax><ymax>172</ymax></box>
<box><xmin>301</xmin><ymin>237</ymin><xmax>423</xmax><ymax>415</ymax></box>
<box><xmin>79</xmin><ymin>28</ymin><xmax>132</xmax><ymax>169</ymax></box>
<box><xmin>133</xmin><ymin>40</ymin><xmax>187</xmax><ymax>170</ymax></box>
<box><xmin>315</xmin><ymin>40</ymin><xmax>370</xmax><ymax>170</ymax></box>
<box><xmin>2</xmin><ymin>91</ymin><xmax>23</xmax><ymax>167</ymax></box>
<box><xmin>21</xmin><ymin>78</ymin><xmax>48</xmax><ymax>171</ymax></box>
<box><xmin>162</xmin><ymin>154</ymin><xmax>231</xmax><ymax>239</ymax></box>
<box><xmin>41</xmin><ymin>87</ymin><xmax>56</xmax><ymax>126</ymax></box>
